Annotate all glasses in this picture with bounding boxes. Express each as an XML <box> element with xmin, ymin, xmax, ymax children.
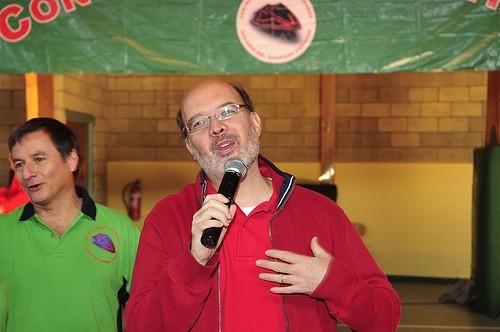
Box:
<box><xmin>185</xmin><ymin>103</ymin><xmax>252</xmax><ymax>133</ymax></box>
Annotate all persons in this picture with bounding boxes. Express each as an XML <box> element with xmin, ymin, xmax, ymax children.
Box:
<box><xmin>0</xmin><ymin>115</ymin><xmax>140</xmax><ymax>332</ymax></box>
<box><xmin>123</xmin><ymin>77</ymin><xmax>403</xmax><ymax>332</ymax></box>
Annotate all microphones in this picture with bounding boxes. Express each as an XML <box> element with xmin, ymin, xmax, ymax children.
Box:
<box><xmin>198</xmin><ymin>156</ymin><xmax>248</xmax><ymax>248</ymax></box>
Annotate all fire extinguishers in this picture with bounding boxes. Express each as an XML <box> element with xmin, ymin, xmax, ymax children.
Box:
<box><xmin>128</xmin><ymin>179</ymin><xmax>142</xmax><ymax>220</ymax></box>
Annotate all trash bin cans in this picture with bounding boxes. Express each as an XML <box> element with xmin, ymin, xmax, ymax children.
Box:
<box><xmin>469</xmin><ymin>146</ymin><xmax>500</xmax><ymax>318</ymax></box>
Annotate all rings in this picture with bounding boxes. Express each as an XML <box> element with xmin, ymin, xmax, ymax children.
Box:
<box><xmin>280</xmin><ymin>274</ymin><xmax>285</xmax><ymax>284</ymax></box>
